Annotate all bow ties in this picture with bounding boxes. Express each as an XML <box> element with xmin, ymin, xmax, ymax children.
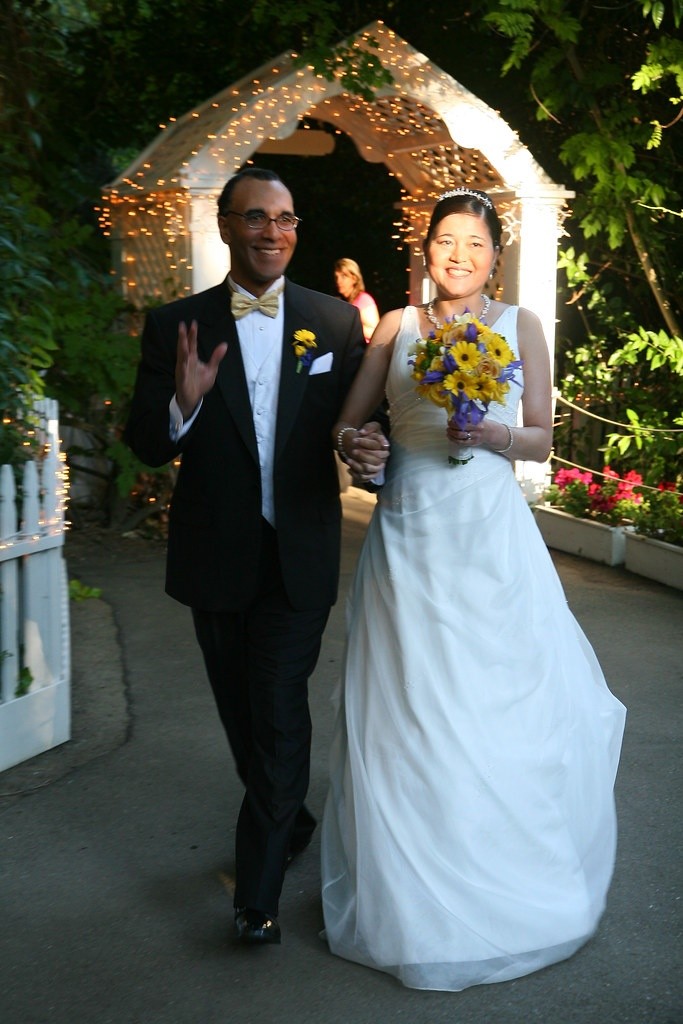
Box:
<box><xmin>227</xmin><ymin>282</ymin><xmax>285</xmax><ymax>320</ymax></box>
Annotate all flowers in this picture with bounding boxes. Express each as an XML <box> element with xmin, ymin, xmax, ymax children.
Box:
<box><xmin>291</xmin><ymin>329</ymin><xmax>317</xmax><ymax>374</ymax></box>
<box><xmin>406</xmin><ymin>307</ymin><xmax>526</xmax><ymax>465</ymax></box>
<box><xmin>541</xmin><ymin>466</ymin><xmax>644</xmax><ymax>525</ymax></box>
<box><xmin>633</xmin><ymin>480</ymin><xmax>683</xmax><ymax>546</ymax></box>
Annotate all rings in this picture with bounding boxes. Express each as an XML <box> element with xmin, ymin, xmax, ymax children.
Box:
<box><xmin>467</xmin><ymin>433</ymin><xmax>472</xmax><ymax>440</ymax></box>
<box><xmin>490</xmin><ymin>423</ymin><xmax>513</xmax><ymax>453</ymax></box>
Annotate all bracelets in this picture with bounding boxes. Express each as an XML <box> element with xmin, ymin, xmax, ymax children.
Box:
<box><xmin>338</xmin><ymin>428</ymin><xmax>358</xmax><ymax>459</ymax></box>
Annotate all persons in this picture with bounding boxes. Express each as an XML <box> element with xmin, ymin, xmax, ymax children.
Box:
<box><xmin>122</xmin><ymin>169</ymin><xmax>391</xmax><ymax>944</ymax></box>
<box><xmin>321</xmin><ymin>188</ymin><xmax>628</xmax><ymax>992</ymax></box>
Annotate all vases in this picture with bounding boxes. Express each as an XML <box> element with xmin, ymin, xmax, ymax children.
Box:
<box><xmin>621</xmin><ymin>526</ymin><xmax>683</xmax><ymax>590</ymax></box>
<box><xmin>535</xmin><ymin>504</ymin><xmax>639</xmax><ymax>566</ymax></box>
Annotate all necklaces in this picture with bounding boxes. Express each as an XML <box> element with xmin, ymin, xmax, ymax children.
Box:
<box><xmin>426</xmin><ymin>294</ymin><xmax>490</xmax><ymax>330</ymax></box>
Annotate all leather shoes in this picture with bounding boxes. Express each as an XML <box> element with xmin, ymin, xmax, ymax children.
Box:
<box><xmin>234</xmin><ymin>907</ymin><xmax>282</xmax><ymax>945</ymax></box>
<box><xmin>284</xmin><ymin>815</ymin><xmax>317</xmax><ymax>871</ymax></box>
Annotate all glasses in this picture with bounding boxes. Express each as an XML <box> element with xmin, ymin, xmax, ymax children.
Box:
<box><xmin>228</xmin><ymin>209</ymin><xmax>302</xmax><ymax>231</ymax></box>
